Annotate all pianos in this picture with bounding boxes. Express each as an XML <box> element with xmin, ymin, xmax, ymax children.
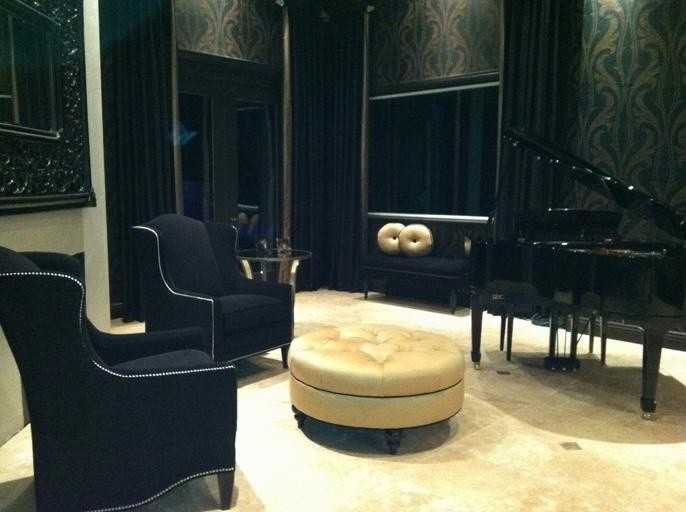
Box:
<box><xmin>467</xmin><ymin>125</ymin><xmax>686</xmax><ymax>419</ymax></box>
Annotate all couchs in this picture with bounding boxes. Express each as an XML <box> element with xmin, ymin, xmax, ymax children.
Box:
<box><xmin>353</xmin><ymin>220</ymin><xmax>488</xmax><ymax>314</ymax></box>
<box><xmin>130</xmin><ymin>214</ymin><xmax>296</xmax><ymax>373</ymax></box>
<box><xmin>6</xmin><ymin>247</ymin><xmax>239</xmax><ymax>512</ymax></box>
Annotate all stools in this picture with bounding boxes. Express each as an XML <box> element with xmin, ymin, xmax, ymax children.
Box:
<box><xmin>285</xmin><ymin>317</ymin><xmax>467</xmax><ymax>456</ymax></box>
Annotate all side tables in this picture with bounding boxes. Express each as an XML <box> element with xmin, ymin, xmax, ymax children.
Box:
<box><xmin>235</xmin><ymin>246</ymin><xmax>314</xmax><ymax>286</ymax></box>
